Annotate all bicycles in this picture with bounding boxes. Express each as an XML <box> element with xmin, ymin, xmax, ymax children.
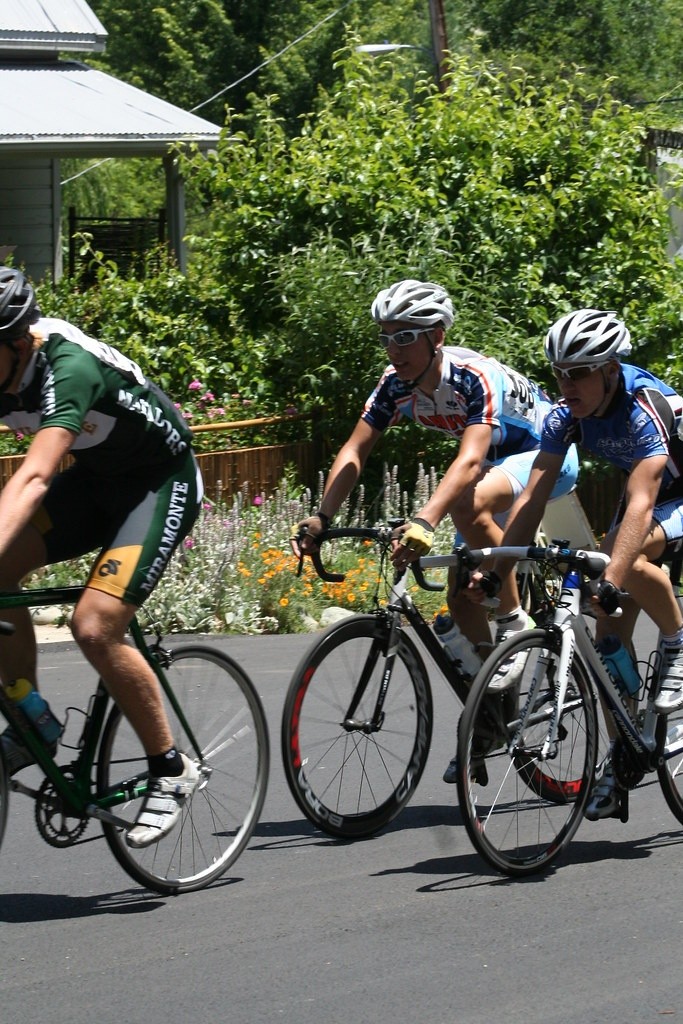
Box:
<box><xmin>281</xmin><ymin>526</ymin><xmax>682</xmax><ymax>875</ymax></box>
<box><xmin>1</xmin><ymin>585</ymin><xmax>271</xmax><ymax>899</ymax></box>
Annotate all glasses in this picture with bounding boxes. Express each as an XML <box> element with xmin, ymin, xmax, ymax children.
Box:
<box><xmin>378</xmin><ymin>327</ymin><xmax>436</xmax><ymax>349</ymax></box>
<box><xmin>550</xmin><ymin>361</ymin><xmax>612</xmax><ymax>380</ymax></box>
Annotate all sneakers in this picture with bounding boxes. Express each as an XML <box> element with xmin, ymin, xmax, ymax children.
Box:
<box><xmin>653</xmin><ymin>645</ymin><xmax>683</xmax><ymax>712</ymax></box>
<box><xmin>484</xmin><ymin>616</ymin><xmax>538</xmax><ymax>695</ymax></box>
<box><xmin>0</xmin><ymin>723</ymin><xmax>56</xmax><ymax>778</ymax></box>
<box><xmin>583</xmin><ymin>755</ymin><xmax>623</xmax><ymax>819</ymax></box>
<box><xmin>125</xmin><ymin>752</ymin><xmax>199</xmax><ymax>848</ymax></box>
<box><xmin>442</xmin><ymin>729</ymin><xmax>508</xmax><ymax>784</ymax></box>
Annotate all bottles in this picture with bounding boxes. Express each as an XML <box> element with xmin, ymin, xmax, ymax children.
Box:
<box><xmin>6</xmin><ymin>678</ymin><xmax>60</xmax><ymax>742</ymax></box>
<box><xmin>434</xmin><ymin>614</ymin><xmax>483</xmax><ymax>678</ymax></box>
<box><xmin>598</xmin><ymin>635</ymin><xmax>640</xmax><ymax>694</ymax></box>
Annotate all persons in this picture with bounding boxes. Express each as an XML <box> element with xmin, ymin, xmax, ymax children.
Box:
<box><xmin>290</xmin><ymin>279</ymin><xmax>578</xmax><ymax>785</ymax></box>
<box><xmin>0</xmin><ymin>264</ymin><xmax>213</xmax><ymax>848</ymax></box>
<box><xmin>469</xmin><ymin>308</ymin><xmax>683</xmax><ymax>821</ymax></box>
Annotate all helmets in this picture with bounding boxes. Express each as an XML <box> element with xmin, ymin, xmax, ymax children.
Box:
<box><xmin>371</xmin><ymin>279</ymin><xmax>454</xmax><ymax>330</ymax></box>
<box><xmin>0</xmin><ymin>264</ymin><xmax>41</xmax><ymax>342</ymax></box>
<box><xmin>543</xmin><ymin>308</ymin><xmax>633</xmax><ymax>362</ymax></box>
